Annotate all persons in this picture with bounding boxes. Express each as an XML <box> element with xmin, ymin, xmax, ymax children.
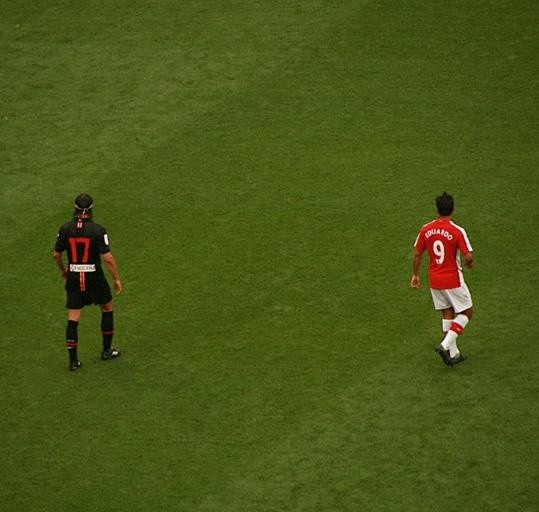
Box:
<box><xmin>50</xmin><ymin>191</ymin><xmax>123</xmax><ymax>372</ymax></box>
<box><xmin>409</xmin><ymin>189</ymin><xmax>474</xmax><ymax>366</ymax></box>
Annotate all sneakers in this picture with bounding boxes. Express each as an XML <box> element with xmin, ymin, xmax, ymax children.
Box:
<box><xmin>69</xmin><ymin>359</ymin><xmax>81</xmax><ymax>371</ymax></box>
<box><xmin>450</xmin><ymin>351</ymin><xmax>467</xmax><ymax>366</ymax></box>
<box><xmin>101</xmin><ymin>349</ymin><xmax>121</xmax><ymax>360</ymax></box>
<box><xmin>435</xmin><ymin>344</ymin><xmax>450</xmax><ymax>365</ymax></box>
